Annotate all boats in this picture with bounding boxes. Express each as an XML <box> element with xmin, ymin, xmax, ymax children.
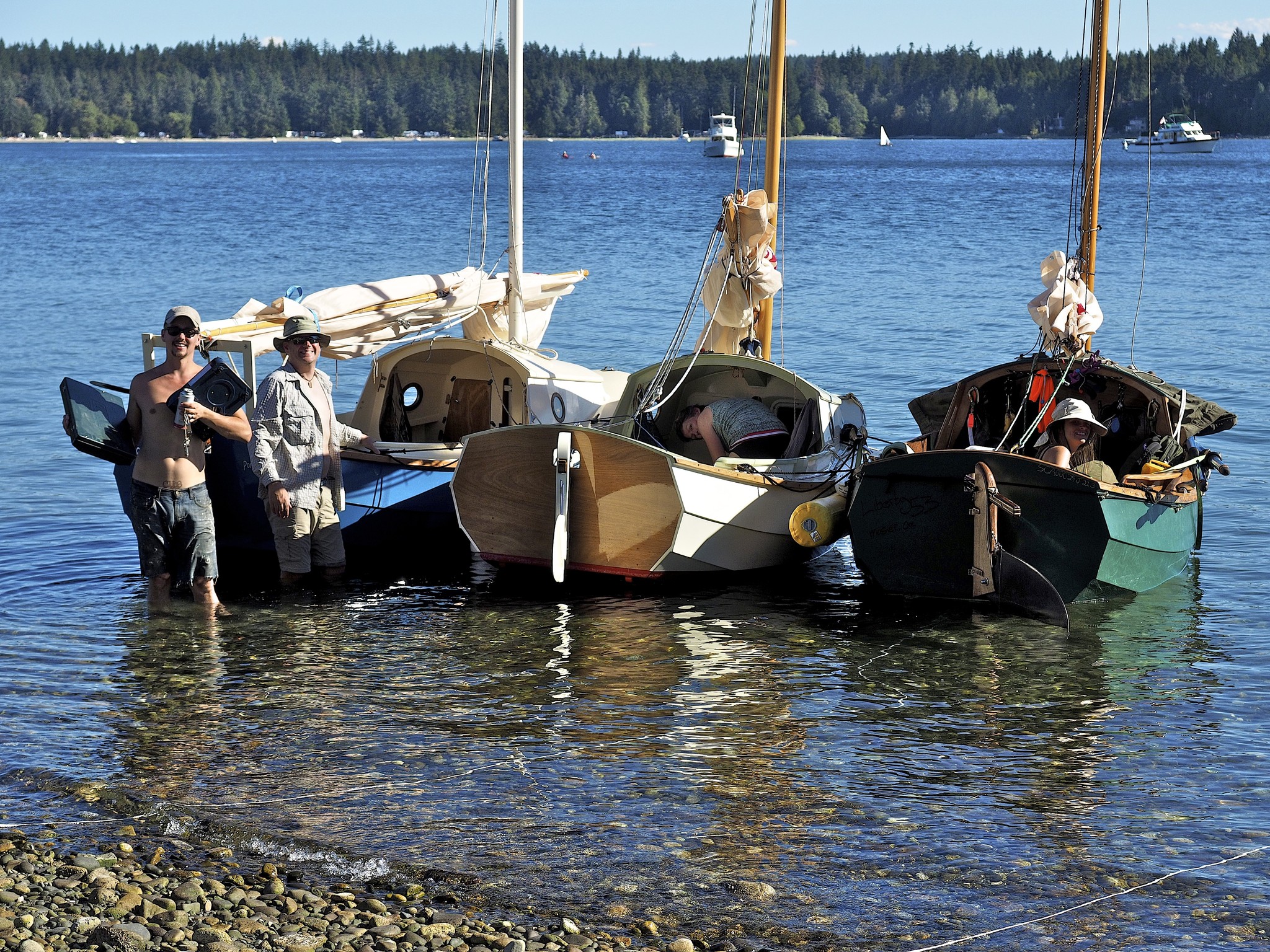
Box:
<box><xmin>1122</xmin><ymin>108</ymin><xmax>1221</xmax><ymax>153</ymax></box>
<box><xmin>703</xmin><ymin>88</ymin><xmax>745</xmax><ymax>157</ymax></box>
<box><xmin>678</xmin><ymin>128</ymin><xmax>691</xmax><ymax>142</ymax></box>
<box><xmin>493</xmin><ymin>135</ymin><xmax>503</xmax><ymax>141</ymax></box>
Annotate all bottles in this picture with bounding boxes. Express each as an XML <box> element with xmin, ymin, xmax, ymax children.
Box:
<box><xmin>174</xmin><ymin>388</ymin><xmax>194</xmax><ymax>430</ymax></box>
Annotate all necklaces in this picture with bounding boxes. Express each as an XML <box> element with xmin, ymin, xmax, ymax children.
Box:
<box><xmin>300</xmin><ymin>374</ymin><xmax>314</xmax><ymax>388</ymax></box>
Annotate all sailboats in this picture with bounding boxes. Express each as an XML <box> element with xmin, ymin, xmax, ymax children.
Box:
<box><xmin>849</xmin><ymin>1</ymin><xmax>1237</xmax><ymax>614</ymax></box>
<box><xmin>112</xmin><ymin>0</ymin><xmax>634</xmax><ymax>582</ymax></box>
<box><xmin>879</xmin><ymin>125</ymin><xmax>891</xmax><ymax>146</ymax></box>
<box><xmin>450</xmin><ymin>0</ymin><xmax>872</xmax><ymax>599</ymax></box>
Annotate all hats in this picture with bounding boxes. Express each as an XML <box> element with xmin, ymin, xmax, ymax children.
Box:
<box><xmin>273</xmin><ymin>315</ymin><xmax>331</xmax><ymax>354</ymax></box>
<box><xmin>1045</xmin><ymin>398</ymin><xmax>1107</xmax><ymax>437</ymax></box>
<box><xmin>163</xmin><ymin>306</ymin><xmax>200</xmax><ymax>330</ymax></box>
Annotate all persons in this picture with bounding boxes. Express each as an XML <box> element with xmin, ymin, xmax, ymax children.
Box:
<box><xmin>562</xmin><ymin>151</ymin><xmax>568</xmax><ymax>159</ymax></box>
<box><xmin>674</xmin><ymin>399</ymin><xmax>790</xmax><ymax>464</ymax></box>
<box><xmin>590</xmin><ymin>152</ymin><xmax>597</xmax><ymax>159</ymax></box>
<box><xmin>1034</xmin><ymin>398</ymin><xmax>1108</xmax><ymax>470</ymax></box>
<box><xmin>247</xmin><ymin>316</ymin><xmax>381</xmax><ymax>589</ymax></box>
<box><xmin>63</xmin><ymin>305</ymin><xmax>251</xmax><ymax>604</ymax></box>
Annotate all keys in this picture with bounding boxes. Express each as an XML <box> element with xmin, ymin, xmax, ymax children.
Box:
<box><xmin>182</xmin><ymin>440</ymin><xmax>190</xmax><ymax>456</ymax></box>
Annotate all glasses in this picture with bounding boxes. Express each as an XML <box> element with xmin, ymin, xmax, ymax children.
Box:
<box><xmin>287</xmin><ymin>336</ymin><xmax>321</xmax><ymax>345</ymax></box>
<box><xmin>165</xmin><ymin>326</ymin><xmax>199</xmax><ymax>338</ymax></box>
